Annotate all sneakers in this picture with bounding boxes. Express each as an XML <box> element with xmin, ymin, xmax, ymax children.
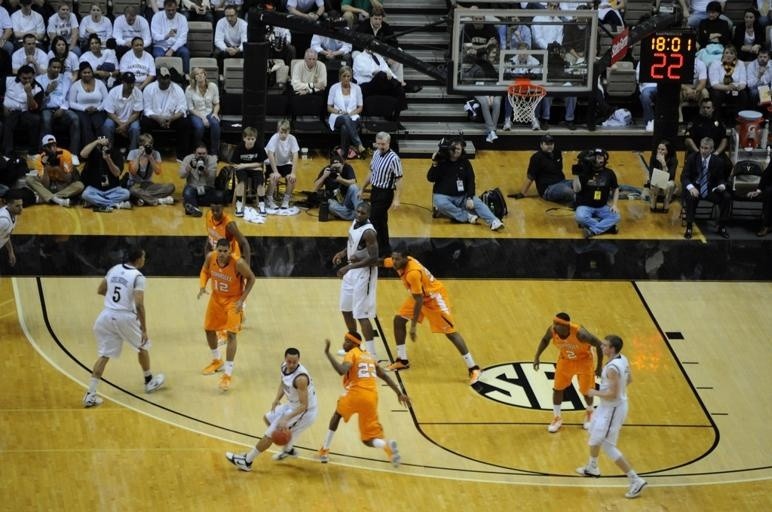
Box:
<box><xmin>490</xmin><ymin>218</ymin><xmax>505</xmax><ymax>230</ymax></box>
<box><xmin>274</xmin><ymin>447</ymin><xmax>332</xmax><ymax>464</ymax></box>
<box><xmin>201</xmin><ymin>359</ymin><xmax>225</xmax><ymax>375</ymax></box>
<box><xmin>547</xmin><ymin>417</ymin><xmax>562</xmax><ymax>433</ymax></box>
<box><xmin>26</xmin><ymin>194</ymin><xmax>296</xmax><ymax>218</ymax></box>
<box><xmin>467</xmin><ymin>213</ymin><xmax>478</xmax><ymax>224</ymax></box>
<box><xmin>82</xmin><ymin>392</ymin><xmax>105</xmax><ymax>409</ymax></box>
<box><xmin>384</xmin><ymin>440</ymin><xmax>400</xmax><ymax>470</ymax></box>
<box><xmin>467</xmin><ymin>366</ymin><xmax>481</xmax><ymax>387</ymax></box>
<box><xmin>623</xmin><ymin>473</ymin><xmax>647</xmax><ymax>498</ymax></box>
<box><xmin>145</xmin><ymin>373</ymin><xmax>167</xmax><ymax>393</ymax></box>
<box><xmin>218</xmin><ymin>373</ymin><xmax>234</xmax><ymax>391</ymax></box>
<box><xmin>575</xmin><ymin>464</ymin><xmax>601</xmax><ymax>476</ymax></box>
<box><xmin>337</xmin><ymin>347</ymin><xmax>410</xmax><ymax>371</ymax></box>
<box><xmin>225</xmin><ymin>451</ymin><xmax>252</xmax><ymax>471</ymax></box>
<box><xmin>582</xmin><ymin>410</ymin><xmax>593</xmax><ymax>430</ymax></box>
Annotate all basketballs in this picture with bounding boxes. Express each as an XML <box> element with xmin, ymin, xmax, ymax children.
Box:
<box><xmin>273</xmin><ymin>427</ymin><xmax>293</xmax><ymax>444</ymax></box>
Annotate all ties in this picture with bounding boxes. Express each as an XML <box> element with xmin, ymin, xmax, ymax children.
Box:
<box><xmin>699</xmin><ymin>158</ymin><xmax>708</xmax><ymax>199</ymax></box>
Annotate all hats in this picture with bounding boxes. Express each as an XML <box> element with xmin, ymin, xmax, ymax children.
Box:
<box><xmin>540</xmin><ymin>134</ymin><xmax>555</xmax><ymax>144</ymax></box>
<box><xmin>157</xmin><ymin>66</ymin><xmax>172</xmax><ymax>77</ymax></box>
<box><xmin>41</xmin><ymin>134</ymin><xmax>58</xmax><ymax>145</ymax></box>
<box><xmin>122</xmin><ymin>72</ymin><xmax>136</xmax><ymax>84</ymax></box>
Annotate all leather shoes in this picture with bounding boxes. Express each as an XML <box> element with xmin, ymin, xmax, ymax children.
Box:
<box><xmin>718</xmin><ymin>227</ymin><xmax>730</xmax><ymax>239</ymax></box>
<box><xmin>684</xmin><ymin>228</ymin><xmax>692</xmax><ymax>239</ymax></box>
<box><xmin>650</xmin><ymin>198</ymin><xmax>669</xmax><ymax>213</ymax></box>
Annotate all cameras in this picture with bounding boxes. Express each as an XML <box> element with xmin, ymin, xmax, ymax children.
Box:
<box><xmin>327</xmin><ymin>167</ymin><xmax>339</xmax><ymax>177</ymax></box>
<box><xmin>194</xmin><ymin>156</ymin><xmax>208</xmax><ymax>171</ymax></box>
<box><xmin>144</xmin><ymin>145</ymin><xmax>153</xmax><ymax>154</ymax></box>
<box><xmin>100</xmin><ymin>144</ymin><xmax>111</xmax><ymax>155</ymax></box>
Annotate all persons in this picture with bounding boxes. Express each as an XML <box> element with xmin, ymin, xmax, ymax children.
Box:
<box><xmin>1</xmin><ymin>1</ymin><xmax>421</xmax><ymax>224</ymax></box>
<box><xmin>531</xmin><ymin>312</ymin><xmax>603</xmax><ymax>436</ymax></box>
<box><xmin>354</xmin><ymin>130</ymin><xmax>402</xmax><ymax>254</ymax></box>
<box><xmin>331</xmin><ymin>202</ymin><xmax>379</xmax><ymax>365</ymax></box>
<box><xmin>79</xmin><ymin>243</ymin><xmax>164</xmax><ymax>407</ymax></box>
<box><xmin>196</xmin><ymin>238</ymin><xmax>255</xmax><ymax>395</ymax></box>
<box><xmin>224</xmin><ymin>346</ymin><xmax>319</xmax><ymax>473</ymax></box>
<box><xmin>348</xmin><ymin>241</ymin><xmax>481</xmax><ymax>386</ymax></box>
<box><xmin>445</xmin><ymin>1</ymin><xmax>771</xmax><ymax>239</ymax></box>
<box><xmin>202</xmin><ymin>198</ymin><xmax>252</xmax><ymax>341</ymax></box>
<box><xmin>574</xmin><ymin>334</ymin><xmax>648</xmax><ymax>500</ymax></box>
<box><xmin>1</xmin><ymin>189</ymin><xmax>24</xmax><ymax>268</ymax></box>
<box><xmin>427</xmin><ymin>134</ymin><xmax>506</xmax><ymax>231</ymax></box>
<box><xmin>314</xmin><ymin>331</ymin><xmax>413</xmax><ymax>469</ymax></box>
